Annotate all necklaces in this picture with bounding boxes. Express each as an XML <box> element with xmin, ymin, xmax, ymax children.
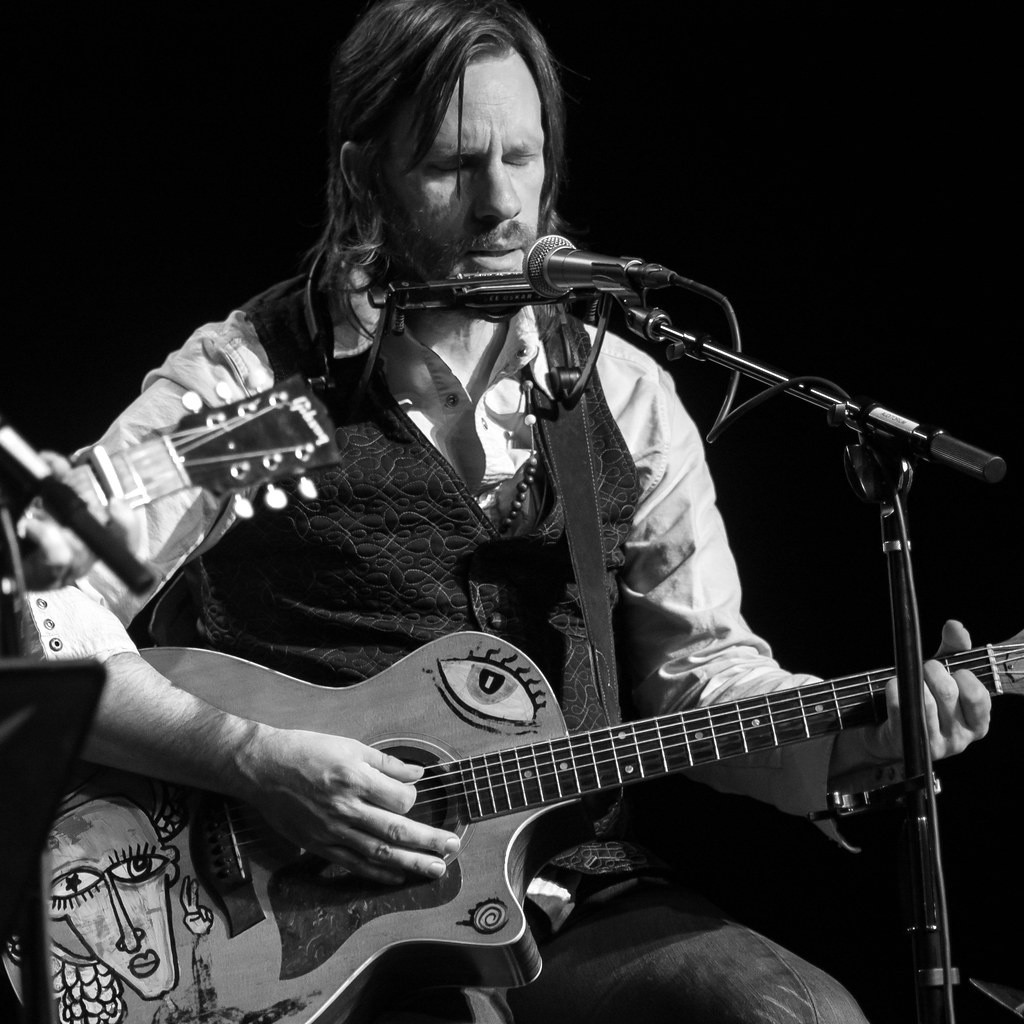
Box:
<box><xmin>498</xmin><ymin>375</ymin><xmax>538</xmax><ymax>535</ymax></box>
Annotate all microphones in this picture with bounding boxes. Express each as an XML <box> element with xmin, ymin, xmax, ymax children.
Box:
<box><xmin>521</xmin><ymin>233</ymin><xmax>678</xmax><ymax>299</ymax></box>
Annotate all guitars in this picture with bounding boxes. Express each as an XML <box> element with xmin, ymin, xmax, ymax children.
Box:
<box><xmin>0</xmin><ymin>373</ymin><xmax>345</xmax><ymax>599</ymax></box>
<box><xmin>0</xmin><ymin>630</ymin><xmax>1023</xmax><ymax>1024</ymax></box>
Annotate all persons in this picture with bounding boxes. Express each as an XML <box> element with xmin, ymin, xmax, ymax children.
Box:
<box><xmin>12</xmin><ymin>1</ymin><xmax>992</xmax><ymax>885</ymax></box>
